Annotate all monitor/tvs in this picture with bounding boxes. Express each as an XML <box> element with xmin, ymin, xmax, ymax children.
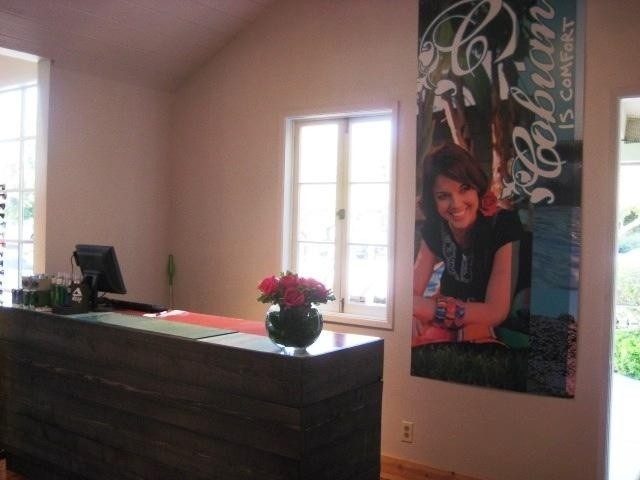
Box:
<box><xmin>75</xmin><ymin>244</ymin><xmax>127</xmax><ymax>311</ymax></box>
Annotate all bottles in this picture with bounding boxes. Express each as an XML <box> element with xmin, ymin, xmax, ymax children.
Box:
<box><xmin>12</xmin><ymin>266</ymin><xmax>83</xmax><ymax>313</ymax></box>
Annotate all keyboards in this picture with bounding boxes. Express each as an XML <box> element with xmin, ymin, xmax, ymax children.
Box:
<box><xmin>108</xmin><ymin>299</ymin><xmax>167</xmax><ymax>313</ymax></box>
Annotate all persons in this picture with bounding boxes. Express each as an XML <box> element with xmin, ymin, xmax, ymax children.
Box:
<box><xmin>409</xmin><ymin>139</ymin><xmax>530</xmax><ymax>351</ymax></box>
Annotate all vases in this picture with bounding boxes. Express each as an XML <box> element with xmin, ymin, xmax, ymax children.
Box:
<box><xmin>265</xmin><ymin>304</ymin><xmax>324</xmax><ymax>353</ymax></box>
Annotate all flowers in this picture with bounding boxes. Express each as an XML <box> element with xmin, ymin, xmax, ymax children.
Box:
<box><xmin>256</xmin><ymin>270</ymin><xmax>335</xmax><ymax>347</ymax></box>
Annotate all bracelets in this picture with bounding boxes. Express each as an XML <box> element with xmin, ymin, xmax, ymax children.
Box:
<box><xmin>428</xmin><ymin>292</ymin><xmax>466</xmax><ymax>333</ymax></box>
